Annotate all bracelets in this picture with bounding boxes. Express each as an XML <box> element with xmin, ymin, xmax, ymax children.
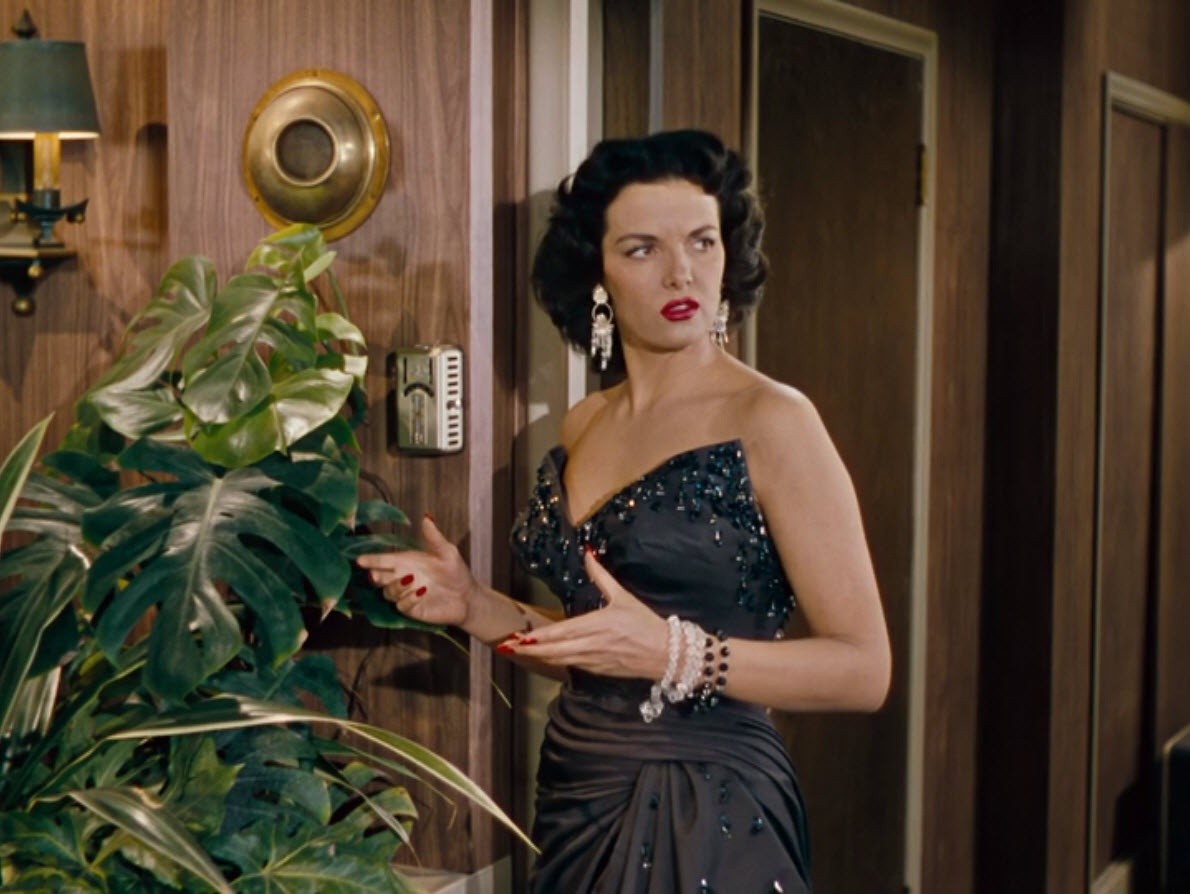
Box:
<box><xmin>639</xmin><ymin>613</ymin><xmax>730</xmax><ymax>725</ymax></box>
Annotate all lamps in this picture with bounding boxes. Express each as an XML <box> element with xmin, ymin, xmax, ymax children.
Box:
<box><xmin>0</xmin><ymin>11</ymin><xmax>101</xmax><ymax>315</ymax></box>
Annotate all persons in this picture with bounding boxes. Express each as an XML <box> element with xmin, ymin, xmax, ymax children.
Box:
<box><xmin>355</xmin><ymin>129</ymin><xmax>892</xmax><ymax>894</ymax></box>
